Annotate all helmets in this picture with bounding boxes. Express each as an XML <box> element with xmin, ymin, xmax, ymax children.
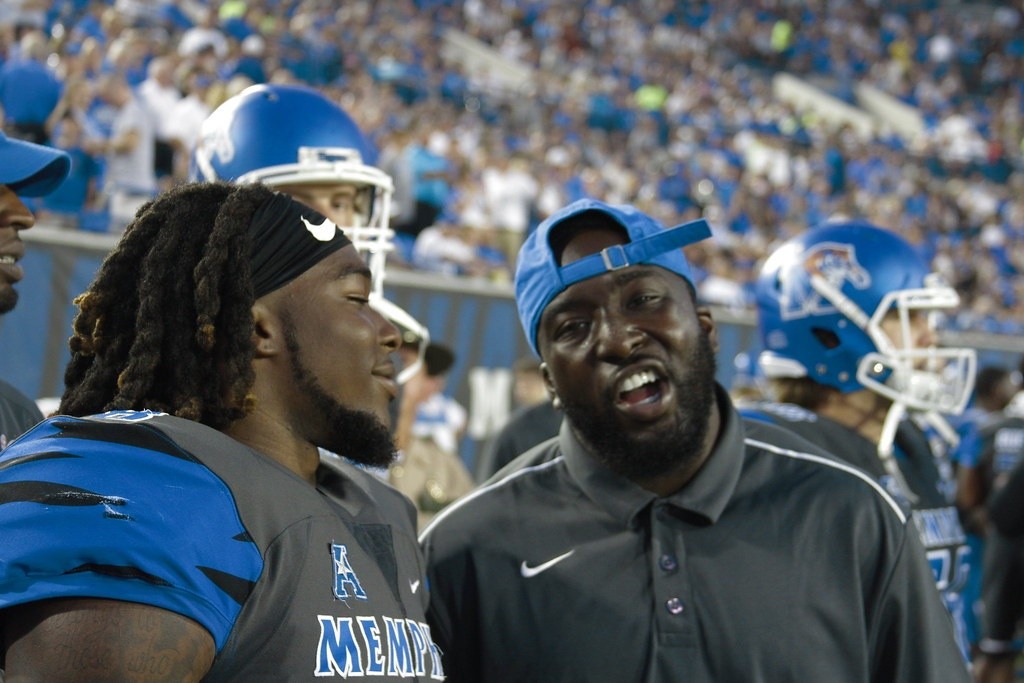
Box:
<box><xmin>754</xmin><ymin>218</ymin><xmax>979</xmax><ymax>416</ymax></box>
<box><xmin>188</xmin><ymin>81</ymin><xmax>396</xmax><ymax>298</ymax></box>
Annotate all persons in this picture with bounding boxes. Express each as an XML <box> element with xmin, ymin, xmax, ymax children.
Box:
<box><xmin>0</xmin><ymin>135</ymin><xmax>72</xmax><ymax>454</ymax></box>
<box><xmin>415</xmin><ymin>200</ymin><xmax>968</xmax><ymax>683</ymax></box>
<box><xmin>478</xmin><ymin>357</ymin><xmax>560</xmax><ymax>486</ymax></box>
<box><xmin>0</xmin><ymin>0</ymin><xmax>1024</xmax><ymax>336</ymax></box>
<box><xmin>729</xmin><ymin>222</ymin><xmax>989</xmax><ymax>683</ymax></box>
<box><xmin>189</xmin><ymin>88</ymin><xmax>390</xmax><ymax>258</ymax></box>
<box><xmin>0</xmin><ymin>181</ymin><xmax>448</xmax><ymax>683</ymax></box>
<box><xmin>391</xmin><ymin>338</ymin><xmax>473</xmax><ymax>520</ymax></box>
<box><xmin>915</xmin><ymin>352</ymin><xmax>1024</xmax><ymax>683</ymax></box>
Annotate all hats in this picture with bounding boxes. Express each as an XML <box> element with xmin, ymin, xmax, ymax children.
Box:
<box><xmin>0</xmin><ymin>127</ymin><xmax>73</xmax><ymax>201</ymax></box>
<box><xmin>512</xmin><ymin>196</ymin><xmax>716</xmax><ymax>365</ymax></box>
